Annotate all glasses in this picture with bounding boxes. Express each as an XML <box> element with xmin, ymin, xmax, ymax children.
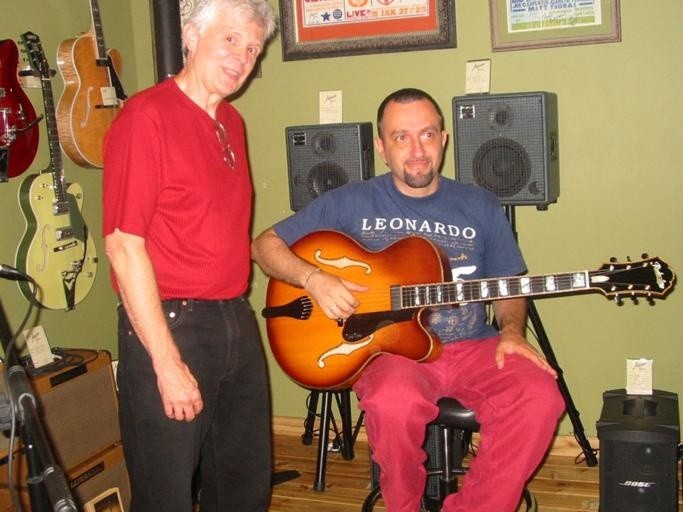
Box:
<box><xmin>215</xmin><ymin>121</ymin><xmax>236</xmax><ymax>169</ymax></box>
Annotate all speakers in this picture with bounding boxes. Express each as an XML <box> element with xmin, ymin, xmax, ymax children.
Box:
<box><xmin>285</xmin><ymin>122</ymin><xmax>375</xmax><ymax>211</ymax></box>
<box><xmin>451</xmin><ymin>91</ymin><xmax>560</xmax><ymax>211</ymax></box>
<box><xmin>595</xmin><ymin>387</ymin><xmax>680</xmax><ymax>512</ymax></box>
<box><xmin>0</xmin><ymin>347</ymin><xmax>132</xmax><ymax>512</ymax></box>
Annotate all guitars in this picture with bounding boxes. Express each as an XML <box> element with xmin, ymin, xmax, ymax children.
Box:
<box><xmin>1</xmin><ymin>38</ymin><xmax>43</xmax><ymax>183</ymax></box>
<box><xmin>56</xmin><ymin>1</ymin><xmax>127</xmax><ymax>168</ymax></box>
<box><xmin>262</xmin><ymin>229</ymin><xmax>677</xmax><ymax>391</ymax></box>
<box><xmin>15</xmin><ymin>30</ymin><xmax>98</xmax><ymax>310</ymax></box>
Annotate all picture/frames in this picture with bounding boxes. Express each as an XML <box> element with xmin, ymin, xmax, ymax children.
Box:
<box><xmin>489</xmin><ymin>0</ymin><xmax>622</xmax><ymax>53</ymax></box>
<box><xmin>279</xmin><ymin>0</ymin><xmax>456</xmax><ymax>62</ymax></box>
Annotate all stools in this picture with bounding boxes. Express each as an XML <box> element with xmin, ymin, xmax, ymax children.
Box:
<box><xmin>361</xmin><ymin>397</ymin><xmax>537</xmax><ymax>512</ymax></box>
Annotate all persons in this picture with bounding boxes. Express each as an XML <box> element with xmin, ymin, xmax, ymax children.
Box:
<box><xmin>250</xmin><ymin>88</ymin><xmax>567</xmax><ymax>512</ymax></box>
<box><xmin>104</xmin><ymin>0</ymin><xmax>278</xmax><ymax>512</ymax></box>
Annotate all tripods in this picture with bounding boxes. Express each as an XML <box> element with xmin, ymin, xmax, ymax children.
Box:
<box><xmin>301</xmin><ymin>389</ymin><xmax>365</xmax><ymax>490</ymax></box>
<box><xmin>462</xmin><ymin>204</ymin><xmax>598</xmax><ymax>467</ymax></box>
